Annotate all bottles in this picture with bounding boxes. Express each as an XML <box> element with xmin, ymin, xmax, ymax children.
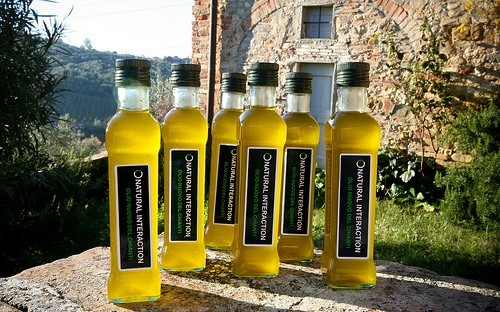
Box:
<box><xmin>106</xmin><ymin>58</ymin><xmax>162</xmax><ymax>304</ymax></box>
<box><xmin>324</xmin><ymin>61</ymin><xmax>382</xmax><ymax>290</ymax></box>
<box><xmin>204</xmin><ymin>62</ymin><xmax>320</xmax><ymax>277</ymax></box>
<box><xmin>162</xmin><ymin>62</ymin><xmax>209</xmax><ymax>272</ymax></box>
